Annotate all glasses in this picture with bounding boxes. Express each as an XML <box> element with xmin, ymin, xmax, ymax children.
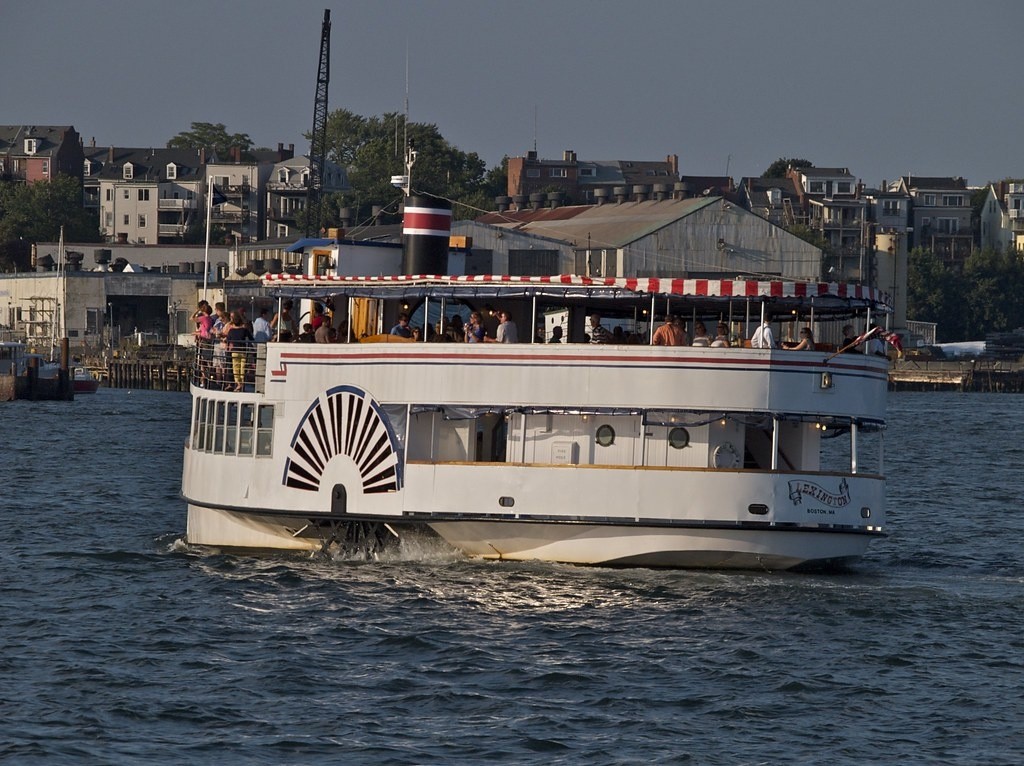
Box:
<box><xmin>716</xmin><ymin>326</ymin><xmax>723</xmax><ymax>328</ymax></box>
<box><xmin>401</xmin><ymin>319</ymin><xmax>408</xmax><ymax>322</ymax></box>
<box><xmin>800</xmin><ymin>331</ymin><xmax>806</xmax><ymax>334</ymax></box>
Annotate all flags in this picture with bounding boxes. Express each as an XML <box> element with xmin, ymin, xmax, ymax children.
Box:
<box><xmin>854</xmin><ymin>327</ymin><xmax>902</xmax><ymax>351</ymax></box>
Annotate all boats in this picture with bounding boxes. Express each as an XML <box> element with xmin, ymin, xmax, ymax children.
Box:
<box><xmin>178</xmin><ymin>174</ymin><xmax>892</xmax><ymax>567</ymax></box>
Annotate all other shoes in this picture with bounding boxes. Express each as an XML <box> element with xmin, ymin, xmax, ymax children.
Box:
<box><xmin>225</xmin><ymin>384</ymin><xmax>234</xmax><ymax>391</ymax></box>
<box><xmin>199</xmin><ymin>380</ymin><xmax>206</xmax><ymax>388</ymax></box>
<box><xmin>234</xmin><ymin>385</ymin><xmax>242</xmax><ymax>392</ymax></box>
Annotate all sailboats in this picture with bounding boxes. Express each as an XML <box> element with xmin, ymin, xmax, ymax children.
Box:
<box><xmin>0</xmin><ymin>224</ymin><xmax>100</xmax><ymax>400</ymax></box>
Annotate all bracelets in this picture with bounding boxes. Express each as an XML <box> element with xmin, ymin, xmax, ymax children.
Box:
<box><xmin>788</xmin><ymin>347</ymin><xmax>789</xmax><ymax>349</ymax></box>
<box><xmin>469</xmin><ymin>333</ymin><xmax>473</xmax><ymax>336</ymax></box>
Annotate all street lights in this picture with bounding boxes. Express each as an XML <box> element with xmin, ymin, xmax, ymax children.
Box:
<box><xmin>108</xmin><ymin>302</ymin><xmax>113</xmax><ymax>352</ymax></box>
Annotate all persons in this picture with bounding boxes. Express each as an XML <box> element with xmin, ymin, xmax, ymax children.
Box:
<box><xmin>782</xmin><ymin>328</ymin><xmax>814</xmax><ymax>351</ymax></box>
<box><xmin>711</xmin><ymin>323</ymin><xmax>730</xmax><ymax>347</ymax></box>
<box><xmin>751</xmin><ymin>314</ymin><xmax>777</xmax><ymax>348</ymax></box>
<box><xmin>675</xmin><ymin>316</ymin><xmax>690</xmax><ymax>346</ymax></box>
<box><xmin>391</xmin><ymin>313</ymin><xmax>465</xmax><ymax>343</ymax></box>
<box><xmin>228</xmin><ymin>312</ymin><xmax>257</xmax><ymax>392</ymax></box>
<box><xmin>314</xmin><ymin>316</ymin><xmax>335</xmax><ymax>343</ymax></box>
<box><xmin>252</xmin><ymin>308</ymin><xmax>274</xmax><ymax>362</ymax></box>
<box><xmin>464</xmin><ymin>312</ymin><xmax>483</xmax><ymax>343</ymax></box>
<box><xmin>189</xmin><ymin>300</ymin><xmax>353</xmax><ymax>392</ymax></box>
<box><xmin>550</xmin><ymin>327</ymin><xmax>562</xmax><ymax>343</ymax></box>
<box><xmin>842</xmin><ymin>325</ymin><xmax>862</xmax><ymax>353</ymax></box>
<box><xmin>692</xmin><ymin>322</ymin><xmax>711</xmax><ymax>346</ymax></box>
<box><xmin>652</xmin><ymin>316</ymin><xmax>688</xmax><ymax>346</ymax></box>
<box><xmin>484</xmin><ymin>312</ymin><xmax>519</xmax><ymax>343</ymax></box>
<box><xmin>868</xmin><ymin>325</ymin><xmax>892</xmax><ymax>361</ymax></box>
<box><xmin>585</xmin><ymin>314</ymin><xmax>643</xmax><ymax>345</ymax></box>
